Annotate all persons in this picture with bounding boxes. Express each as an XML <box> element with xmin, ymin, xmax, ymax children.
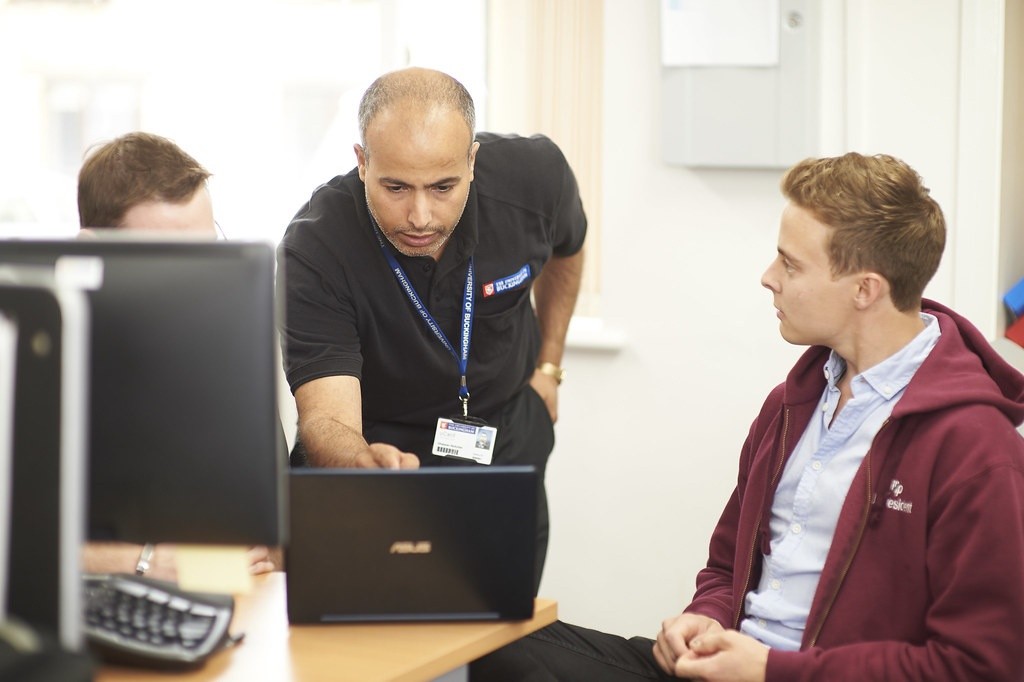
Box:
<box><xmin>273</xmin><ymin>67</ymin><xmax>588</xmax><ymax>600</ymax></box>
<box><xmin>468</xmin><ymin>153</ymin><xmax>1024</xmax><ymax>680</ymax></box>
<box><xmin>76</xmin><ymin>132</ymin><xmax>290</xmax><ymax>587</ymax></box>
<box><xmin>477</xmin><ymin>431</ymin><xmax>489</xmax><ymax>449</ymax></box>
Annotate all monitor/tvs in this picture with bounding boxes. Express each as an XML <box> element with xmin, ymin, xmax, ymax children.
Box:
<box><xmin>0</xmin><ymin>236</ymin><xmax>286</xmax><ymax>682</ymax></box>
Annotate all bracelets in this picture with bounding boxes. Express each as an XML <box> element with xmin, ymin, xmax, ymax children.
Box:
<box><xmin>134</xmin><ymin>540</ymin><xmax>153</xmax><ymax>578</ymax></box>
<box><xmin>539</xmin><ymin>361</ymin><xmax>563</xmax><ymax>385</ymax></box>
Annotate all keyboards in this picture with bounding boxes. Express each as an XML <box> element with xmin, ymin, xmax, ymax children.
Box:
<box><xmin>81</xmin><ymin>573</ymin><xmax>234</xmax><ymax>673</ymax></box>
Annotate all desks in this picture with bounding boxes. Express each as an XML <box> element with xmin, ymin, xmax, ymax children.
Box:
<box><xmin>80</xmin><ymin>537</ymin><xmax>560</xmax><ymax>682</ymax></box>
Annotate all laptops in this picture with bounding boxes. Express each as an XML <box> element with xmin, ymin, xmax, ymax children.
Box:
<box><xmin>281</xmin><ymin>465</ymin><xmax>538</xmax><ymax>626</ymax></box>
